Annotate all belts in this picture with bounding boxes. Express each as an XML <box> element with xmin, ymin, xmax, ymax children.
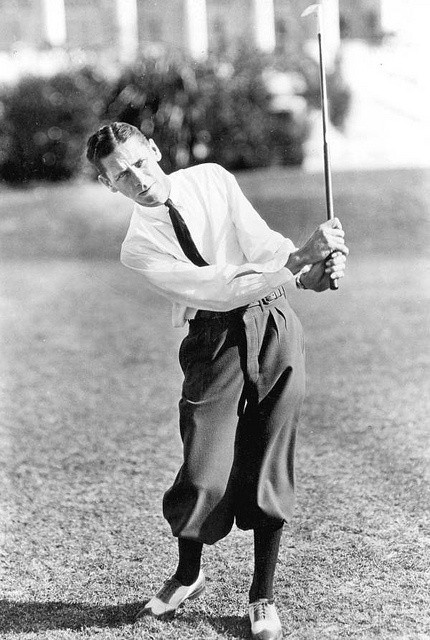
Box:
<box><xmin>189</xmin><ymin>284</ymin><xmax>286</xmax><ymax>318</ymax></box>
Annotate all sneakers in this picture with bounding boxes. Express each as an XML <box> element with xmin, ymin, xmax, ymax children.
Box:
<box><xmin>137</xmin><ymin>571</ymin><xmax>205</xmax><ymax>623</ymax></box>
<box><xmin>248</xmin><ymin>599</ymin><xmax>283</xmax><ymax>636</ymax></box>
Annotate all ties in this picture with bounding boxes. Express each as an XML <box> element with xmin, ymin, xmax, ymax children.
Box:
<box><xmin>164</xmin><ymin>199</ymin><xmax>209</xmax><ymax>267</ymax></box>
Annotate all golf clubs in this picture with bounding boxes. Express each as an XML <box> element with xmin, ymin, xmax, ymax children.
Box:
<box><xmin>300</xmin><ymin>4</ymin><xmax>339</xmax><ymax>290</ymax></box>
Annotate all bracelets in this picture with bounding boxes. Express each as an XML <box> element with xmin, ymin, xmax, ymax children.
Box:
<box><xmin>296</xmin><ymin>273</ymin><xmax>307</xmax><ymax>291</ymax></box>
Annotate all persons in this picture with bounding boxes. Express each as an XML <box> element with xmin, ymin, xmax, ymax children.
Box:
<box><xmin>84</xmin><ymin>119</ymin><xmax>352</xmax><ymax>640</ymax></box>
